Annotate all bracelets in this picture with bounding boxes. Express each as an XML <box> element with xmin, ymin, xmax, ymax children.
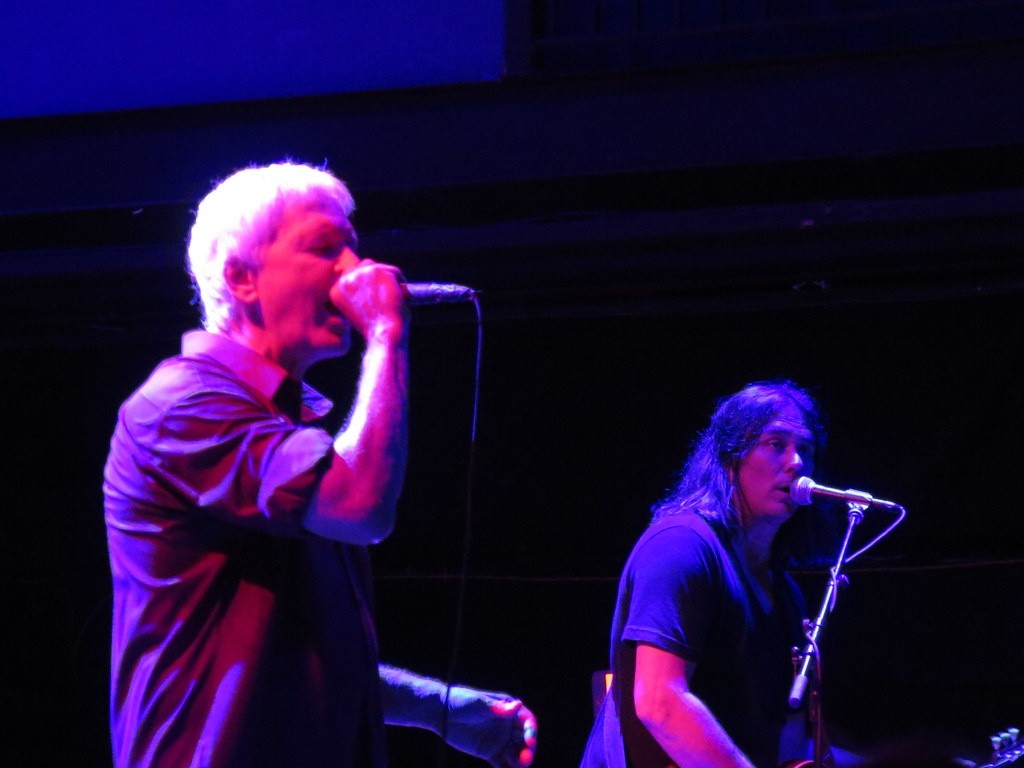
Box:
<box><xmin>441</xmin><ymin>686</ymin><xmax>451</xmax><ymax>741</ymax></box>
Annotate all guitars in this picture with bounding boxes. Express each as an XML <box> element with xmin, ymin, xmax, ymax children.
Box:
<box><xmin>664</xmin><ymin>724</ymin><xmax>1024</xmax><ymax>768</ymax></box>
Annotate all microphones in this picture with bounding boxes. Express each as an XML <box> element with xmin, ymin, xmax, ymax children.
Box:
<box><xmin>395</xmin><ymin>280</ymin><xmax>475</xmax><ymax>306</ymax></box>
<box><xmin>789</xmin><ymin>475</ymin><xmax>902</xmax><ymax>512</ymax></box>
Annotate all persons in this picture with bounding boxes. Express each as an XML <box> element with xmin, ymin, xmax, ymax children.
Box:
<box><xmin>578</xmin><ymin>379</ymin><xmax>978</xmax><ymax>767</ymax></box>
<box><xmin>104</xmin><ymin>161</ymin><xmax>539</xmax><ymax>768</ymax></box>
